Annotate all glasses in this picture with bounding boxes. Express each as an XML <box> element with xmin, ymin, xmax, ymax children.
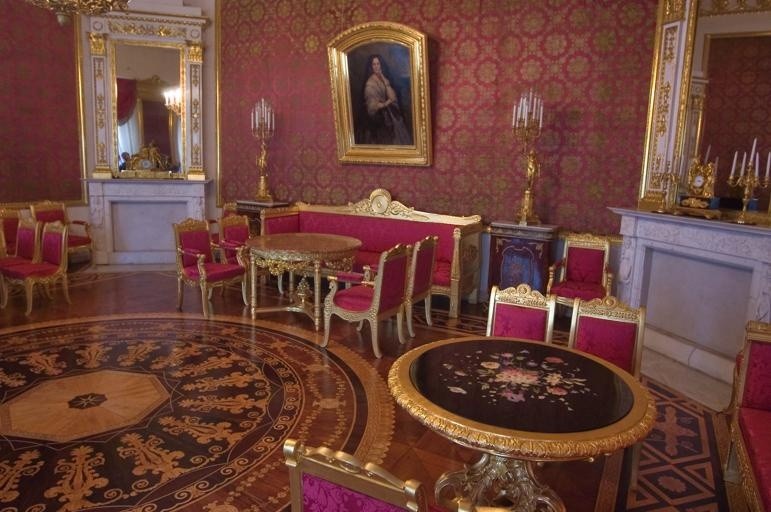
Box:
<box><xmin>512</xmin><ymin>90</ymin><xmax>544</xmax><ymax>227</ymax></box>
<box><xmin>251</xmin><ymin>97</ymin><xmax>275</xmax><ymax>205</ymax></box>
<box><xmin>729</xmin><ymin>136</ymin><xmax>771</xmax><ymax>226</ymax></box>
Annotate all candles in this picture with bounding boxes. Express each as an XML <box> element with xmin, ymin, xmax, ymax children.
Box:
<box><xmin>705</xmin><ymin>142</ymin><xmax>719</xmax><ymax>176</ymax></box>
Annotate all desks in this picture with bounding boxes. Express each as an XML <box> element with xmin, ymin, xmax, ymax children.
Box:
<box><xmin>245</xmin><ymin>232</ymin><xmax>362</xmax><ymax>331</ymax></box>
<box><xmin>387</xmin><ymin>336</ymin><xmax>657</xmax><ymax>512</ymax></box>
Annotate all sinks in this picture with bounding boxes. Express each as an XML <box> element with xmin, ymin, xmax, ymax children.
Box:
<box><xmin>723</xmin><ymin>317</ymin><xmax>771</xmax><ymax>510</ymax></box>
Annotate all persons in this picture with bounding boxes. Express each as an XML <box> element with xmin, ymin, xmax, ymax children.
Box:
<box><xmin>364</xmin><ymin>55</ymin><xmax>408</xmax><ymax>144</ymax></box>
<box><xmin>118</xmin><ymin>152</ymin><xmax>130</xmax><ymax>172</ymax></box>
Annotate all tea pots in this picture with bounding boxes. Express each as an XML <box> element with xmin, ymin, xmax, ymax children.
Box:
<box><xmin>638</xmin><ymin>0</ymin><xmax>771</xmax><ymax>216</ymax></box>
<box><xmin>74</xmin><ymin>11</ymin><xmax>210</xmax><ymax>179</ymax></box>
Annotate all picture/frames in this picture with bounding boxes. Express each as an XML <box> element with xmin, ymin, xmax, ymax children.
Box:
<box><xmin>325</xmin><ymin>21</ymin><xmax>434</xmax><ymax>168</ymax></box>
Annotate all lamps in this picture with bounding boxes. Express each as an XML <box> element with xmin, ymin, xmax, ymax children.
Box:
<box><xmin>0</xmin><ymin>200</ymin><xmax>92</xmax><ymax>317</ymax></box>
<box><xmin>729</xmin><ymin>136</ymin><xmax>771</xmax><ymax>226</ymax></box>
<box><xmin>251</xmin><ymin>97</ymin><xmax>275</xmax><ymax>205</ymax></box>
<box><xmin>512</xmin><ymin>90</ymin><xmax>544</xmax><ymax>227</ymax></box>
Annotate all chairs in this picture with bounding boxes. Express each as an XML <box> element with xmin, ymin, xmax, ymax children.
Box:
<box><xmin>218</xmin><ymin>209</ymin><xmax>285</xmax><ymax>303</ymax></box>
<box><xmin>0</xmin><ymin>200</ymin><xmax>92</xmax><ymax>317</ymax></box>
<box><xmin>547</xmin><ymin>233</ymin><xmax>615</xmax><ymax>323</ymax></box>
<box><xmin>320</xmin><ymin>243</ymin><xmax>408</xmax><ymax>358</ymax></box>
<box><xmin>362</xmin><ymin>235</ymin><xmax>440</xmax><ymax>337</ymax></box>
<box><xmin>485</xmin><ymin>283</ymin><xmax>557</xmax><ymax>343</ymax></box>
<box><xmin>568</xmin><ymin>296</ymin><xmax>646</xmax><ymax>381</ymax></box>
<box><xmin>284</xmin><ymin>438</ymin><xmax>430</xmax><ymax>512</ymax></box>
<box><xmin>174</xmin><ymin>219</ymin><xmax>250</xmax><ymax>319</ymax></box>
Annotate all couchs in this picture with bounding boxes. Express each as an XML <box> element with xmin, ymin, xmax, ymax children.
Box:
<box><xmin>259</xmin><ymin>189</ymin><xmax>483</xmax><ymax>319</ymax></box>
<box><xmin>723</xmin><ymin>317</ymin><xmax>771</xmax><ymax>510</ymax></box>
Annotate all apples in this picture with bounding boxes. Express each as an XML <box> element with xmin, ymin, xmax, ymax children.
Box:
<box><xmin>705</xmin><ymin>142</ymin><xmax>719</xmax><ymax>176</ymax></box>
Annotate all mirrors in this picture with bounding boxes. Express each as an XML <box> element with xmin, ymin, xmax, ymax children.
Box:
<box><xmin>74</xmin><ymin>11</ymin><xmax>210</xmax><ymax>179</ymax></box>
<box><xmin>638</xmin><ymin>0</ymin><xmax>771</xmax><ymax>216</ymax></box>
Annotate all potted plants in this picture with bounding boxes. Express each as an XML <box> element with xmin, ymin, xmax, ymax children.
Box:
<box><xmin>325</xmin><ymin>21</ymin><xmax>434</xmax><ymax>168</ymax></box>
